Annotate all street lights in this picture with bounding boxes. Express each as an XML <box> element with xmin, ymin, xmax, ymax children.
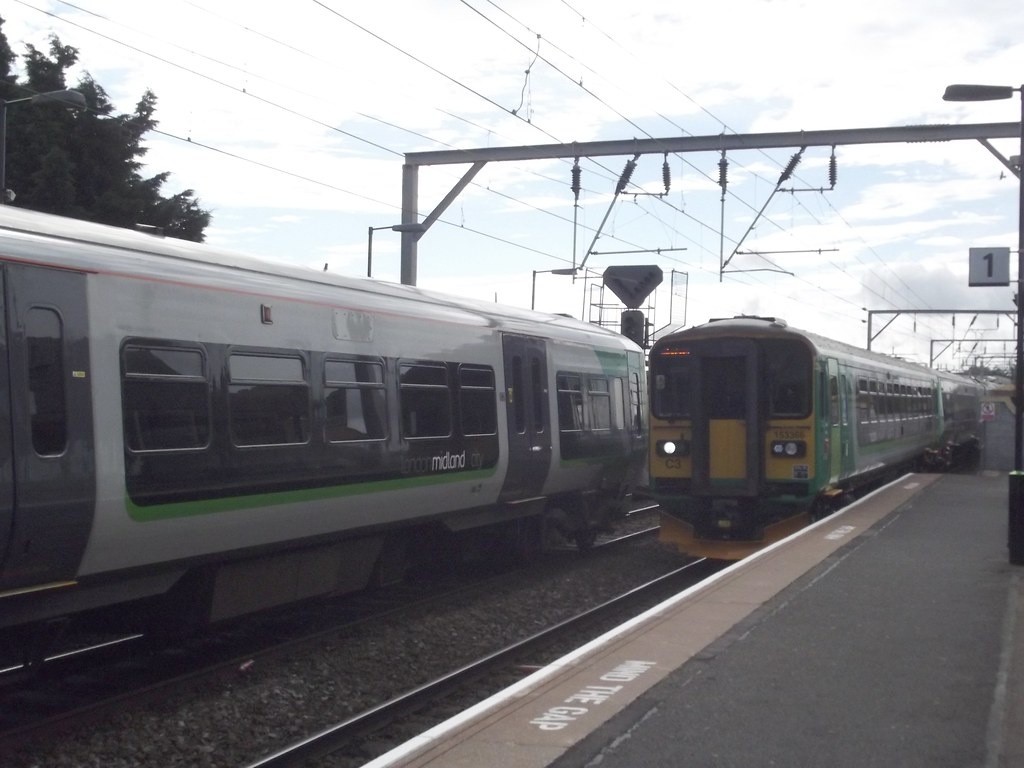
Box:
<box><xmin>938</xmin><ymin>80</ymin><xmax>1023</xmax><ymax>565</ymax></box>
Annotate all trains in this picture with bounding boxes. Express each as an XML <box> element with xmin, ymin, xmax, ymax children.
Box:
<box><xmin>649</xmin><ymin>320</ymin><xmax>1023</xmax><ymax>559</ymax></box>
<box><xmin>0</xmin><ymin>198</ymin><xmax>650</xmax><ymax>712</ymax></box>
